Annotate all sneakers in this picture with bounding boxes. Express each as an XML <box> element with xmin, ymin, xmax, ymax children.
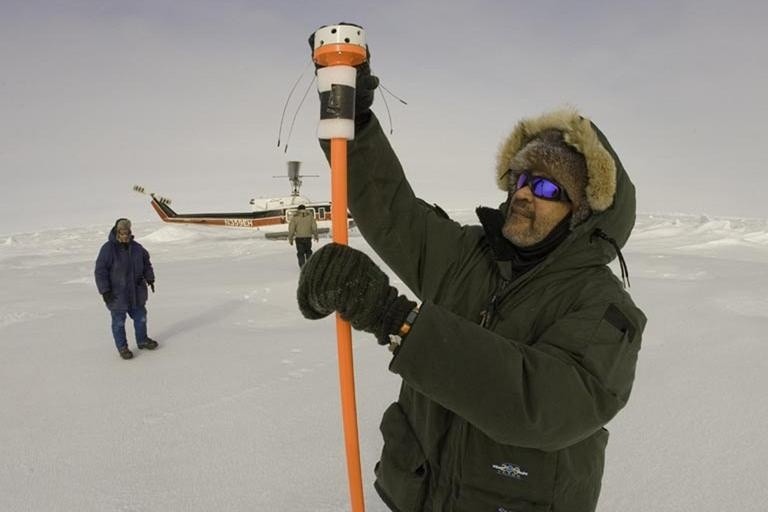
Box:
<box><xmin>137</xmin><ymin>336</ymin><xmax>158</xmax><ymax>350</ymax></box>
<box><xmin>118</xmin><ymin>348</ymin><xmax>135</xmax><ymax>361</ymax></box>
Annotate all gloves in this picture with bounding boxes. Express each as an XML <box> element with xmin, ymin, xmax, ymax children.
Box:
<box><xmin>296</xmin><ymin>240</ymin><xmax>419</xmax><ymax>347</ymax></box>
<box><xmin>307</xmin><ymin>21</ymin><xmax>379</xmax><ymax>112</ymax></box>
<box><xmin>147</xmin><ymin>282</ymin><xmax>155</xmax><ymax>293</ymax></box>
<box><xmin>103</xmin><ymin>292</ymin><xmax>120</xmax><ymax>305</ymax></box>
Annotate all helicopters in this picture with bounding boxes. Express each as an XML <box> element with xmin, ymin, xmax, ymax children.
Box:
<box><xmin>132</xmin><ymin>161</ymin><xmax>359</xmax><ymax>241</ymax></box>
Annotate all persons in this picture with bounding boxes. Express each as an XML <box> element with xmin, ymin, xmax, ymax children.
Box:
<box><xmin>95</xmin><ymin>219</ymin><xmax>159</xmax><ymax>359</ymax></box>
<box><xmin>288</xmin><ymin>204</ymin><xmax>320</xmax><ymax>269</ymax></box>
<box><xmin>296</xmin><ymin>23</ymin><xmax>650</xmax><ymax>512</ymax></box>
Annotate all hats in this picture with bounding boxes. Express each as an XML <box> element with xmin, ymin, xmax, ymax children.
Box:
<box><xmin>115</xmin><ymin>219</ymin><xmax>132</xmax><ymax>231</ymax></box>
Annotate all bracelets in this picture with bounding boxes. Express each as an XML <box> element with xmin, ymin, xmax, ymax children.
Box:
<box><xmin>388</xmin><ymin>306</ymin><xmax>423</xmax><ymax>353</ymax></box>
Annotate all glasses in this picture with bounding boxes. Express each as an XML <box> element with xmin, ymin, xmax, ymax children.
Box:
<box><xmin>514</xmin><ymin>171</ymin><xmax>569</xmax><ymax>206</ymax></box>
